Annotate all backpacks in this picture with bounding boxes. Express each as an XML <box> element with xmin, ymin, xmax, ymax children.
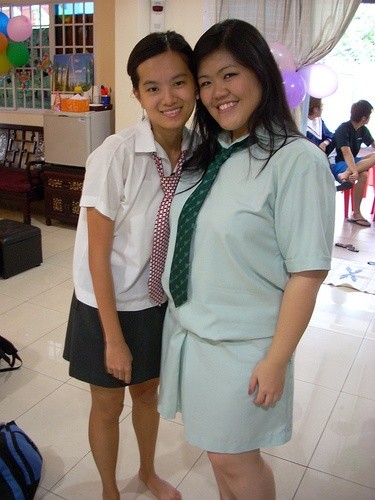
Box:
<box><xmin>0</xmin><ymin>420</ymin><xmax>42</xmax><ymax>500</ymax></box>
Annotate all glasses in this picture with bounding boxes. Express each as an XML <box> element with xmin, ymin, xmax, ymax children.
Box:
<box><xmin>315</xmin><ymin>103</ymin><xmax>324</xmax><ymax>110</ymax></box>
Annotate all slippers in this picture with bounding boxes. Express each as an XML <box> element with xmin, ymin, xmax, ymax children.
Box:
<box><xmin>347</xmin><ymin>218</ymin><xmax>371</xmax><ymax>227</ymax></box>
<box><xmin>336</xmin><ymin>178</ymin><xmax>352</xmax><ymax>191</ymax></box>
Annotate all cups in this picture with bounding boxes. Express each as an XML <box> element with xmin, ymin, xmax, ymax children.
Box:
<box><xmin>101</xmin><ymin>95</ymin><xmax>110</xmax><ymax>109</ymax></box>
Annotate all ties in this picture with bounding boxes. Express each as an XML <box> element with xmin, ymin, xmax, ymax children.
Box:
<box><xmin>148</xmin><ymin>150</ymin><xmax>189</xmax><ymax>307</ymax></box>
<box><xmin>169</xmin><ymin>135</ymin><xmax>258</xmax><ymax>309</ymax></box>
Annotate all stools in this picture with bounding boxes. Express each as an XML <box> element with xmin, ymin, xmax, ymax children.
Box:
<box><xmin>0</xmin><ymin>218</ymin><xmax>43</xmax><ymax>279</ymax></box>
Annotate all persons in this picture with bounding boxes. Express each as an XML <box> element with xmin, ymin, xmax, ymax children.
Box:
<box><xmin>157</xmin><ymin>18</ymin><xmax>336</xmax><ymax>500</ymax></box>
<box><xmin>306</xmin><ymin>96</ymin><xmax>335</xmax><ymax>158</ymax></box>
<box><xmin>329</xmin><ymin>99</ymin><xmax>375</xmax><ymax>225</ymax></box>
<box><xmin>63</xmin><ymin>30</ymin><xmax>197</xmax><ymax>499</ymax></box>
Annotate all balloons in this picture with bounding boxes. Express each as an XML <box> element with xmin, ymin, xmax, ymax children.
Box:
<box><xmin>268</xmin><ymin>43</ymin><xmax>339</xmax><ymax>107</ymax></box>
<box><xmin>0</xmin><ymin>13</ymin><xmax>33</xmax><ymax>76</ymax></box>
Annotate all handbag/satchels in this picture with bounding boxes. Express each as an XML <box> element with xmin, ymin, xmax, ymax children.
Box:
<box><xmin>0</xmin><ymin>335</ymin><xmax>23</xmax><ymax>372</ymax></box>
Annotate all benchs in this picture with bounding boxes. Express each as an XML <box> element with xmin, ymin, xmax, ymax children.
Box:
<box><xmin>0</xmin><ymin>123</ymin><xmax>44</xmax><ymax>226</ymax></box>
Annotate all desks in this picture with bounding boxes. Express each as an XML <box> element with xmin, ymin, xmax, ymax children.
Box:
<box><xmin>43</xmin><ymin>163</ymin><xmax>86</xmax><ymax>226</ymax></box>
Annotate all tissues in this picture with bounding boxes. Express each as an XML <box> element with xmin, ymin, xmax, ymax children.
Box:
<box><xmin>60</xmin><ymin>94</ymin><xmax>90</xmax><ymax>112</ymax></box>
